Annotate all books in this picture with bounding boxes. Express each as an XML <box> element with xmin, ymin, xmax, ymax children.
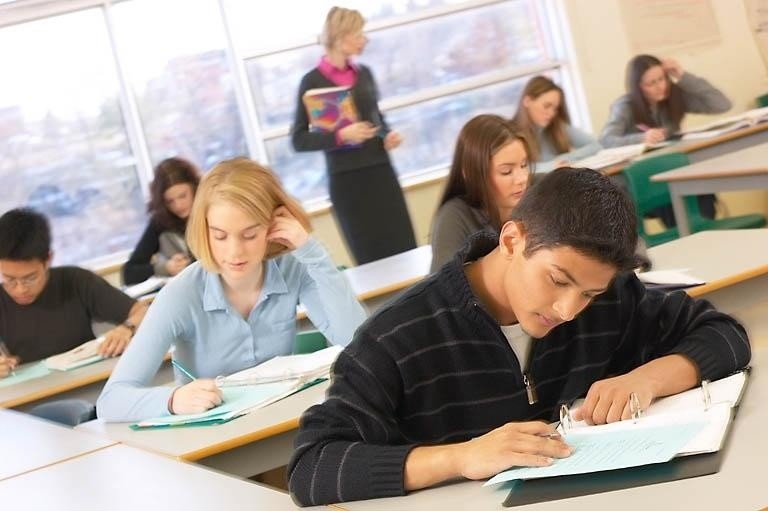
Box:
<box><xmin>130</xmin><ymin>342</ymin><xmax>349</xmax><ymax>430</ymax></box>
<box><xmin>573</xmin><ymin>107</ymin><xmax>768</xmax><ymax>172</ymax></box>
<box><xmin>42</xmin><ymin>333</ymin><xmax>111</xmax><ymax>372</ymax></box>
<box><xmin>635</xmin><ymin>270</ymin><xmax>700</xmax><ymax>294</ymax></box>
<box><xmin>303</xmin><ymin>85</ymin><xmax>362</xmax><ymax>153</ymax></box>
<box><xmin>483</xmin><ymin>364</ymin><xmax>750</xmax><ymax>487</ymax></box>
<box><xmin>121</xmin><ymin>273</ymin><xmax>177</xmax><ymax>301</ymax></box>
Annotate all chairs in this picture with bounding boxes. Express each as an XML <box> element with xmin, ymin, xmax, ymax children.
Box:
<box><xmin>756</xmin><ymin>94</ymin><xmax>768</xmax><ymax>107</ymax></box>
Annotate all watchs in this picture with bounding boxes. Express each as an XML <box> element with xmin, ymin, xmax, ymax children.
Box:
<box><xmin>122</xmin><ymin>319</ymin><xmax>136</xmax><ymax>338</ymax></box>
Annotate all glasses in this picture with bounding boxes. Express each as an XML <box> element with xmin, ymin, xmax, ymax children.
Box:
<box><xmin>0</xmin><ymin>263</ymin><xmax>47</xmax><ymax>288</ymax></box>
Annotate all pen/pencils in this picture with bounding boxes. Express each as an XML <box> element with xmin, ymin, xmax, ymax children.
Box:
<box><xmin>636</xmin><ymin>123</ymin><xmax>650</xmax><ymax>132</ymax></box>
<box><xmin>171</xmin><ymin>359</ymin><xmax>225</xmax><ymax>404</ymax></box>
<box><xmin>536</xmin><ymin>432</ymin><xmax>560</xmax><ymax>438</ymax></box>
<box><xmin>0</xmin><ymin>341</ymin><xmax>15</xmax><ymax>377</ymax></box>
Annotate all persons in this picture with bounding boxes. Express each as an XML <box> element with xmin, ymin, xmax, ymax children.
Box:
<box><xmin>120</xmin><ymin>157</ymin><xmax>201</xmax><ymax>287</ymax></box>
<box><xmin>97</xmin><ymin>155</ymin><xmax>370</xmax><ymax>423</ymax></box>
<box><xmin>424</xmin><ymin>113</ymin><xmax>551</xmax><ymax>276</ymax></box>
<box><xmin>289</xmin><ymin>5</ymin><xmax>416</xmax><ymax>269</ymax></box>
<box><xmin>0</xmin><ymin>205</ymin><xmax>149</xmax><ymax>381</ymax></box>
<box><xmin>599</xmin><ymin>54</ymin><xmax>731</xmax><ymax>231</ymax></box>
<box><xmin>509</xmin><ymin>74</ymin><xmax>602</xmax><ymax>181</ymax></box>
<box><xmin>284</xmin><ymin>165</ymin><xmax>752</xmax><ymax>509</ymax></box>
